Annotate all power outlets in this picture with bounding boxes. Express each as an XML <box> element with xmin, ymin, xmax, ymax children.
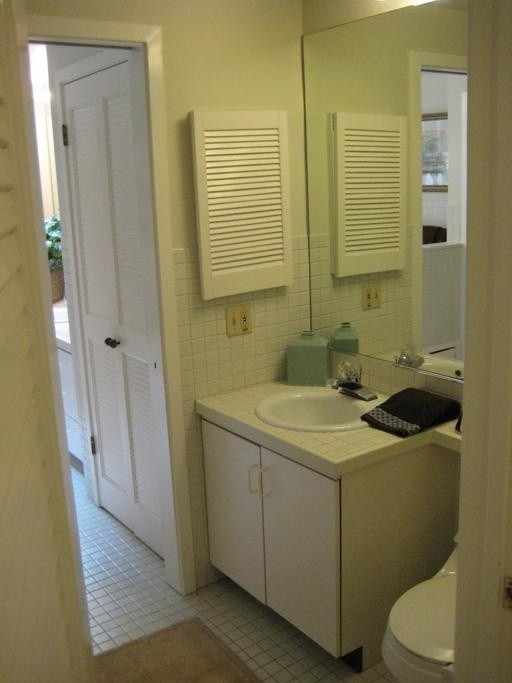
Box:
<box><xmin>225</xmin><ymin>303</ymin><xmax>252</xmax><ymax>336</ymax></box>
<box><xmin>361</xmin><ymin>281</ymin><xmax>380</xmax><ymax>311</ymax></box>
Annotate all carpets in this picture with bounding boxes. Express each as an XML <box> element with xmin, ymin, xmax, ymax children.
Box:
<box><xmin>95</xmin><ymin>618</ymin><xmax>263</xmax><ymax>683</ymax></box>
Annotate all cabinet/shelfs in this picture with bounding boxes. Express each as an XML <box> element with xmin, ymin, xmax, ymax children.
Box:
<box><xmin>202</xmin><ymin>418</ymin><xmax>461</xmax><ymax>671</ymax></box>
<box><xmin>62</xmin><ymin>60</ymin><xmax>176</xmax><ymax>561</ymax></box>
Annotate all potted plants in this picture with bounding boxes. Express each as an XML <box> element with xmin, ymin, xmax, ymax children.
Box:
<box><xmin>43</xmin><ymin>213</ymin><xmax>64</xmax><ymax>302</ymax></box>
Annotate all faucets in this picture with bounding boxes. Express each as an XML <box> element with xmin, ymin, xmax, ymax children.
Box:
<box><xmin>399</xmin><ymin>354</ymin><xmax>424</xmax><ymax>368</ymax></box>
<box><xmin>332</xmin><ymin>360</ymin><xmax>377</xmax><ymax>400</ymax></box>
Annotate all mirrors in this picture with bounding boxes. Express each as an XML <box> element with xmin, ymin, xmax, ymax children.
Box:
<box><xmin>302</xmin><ymin>0</ymin><xmax>469</xmax><ymax>379</ymax></box>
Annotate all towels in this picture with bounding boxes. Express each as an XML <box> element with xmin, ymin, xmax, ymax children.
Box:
<box><xmin>361</xmin><ymin>388</ymin><xmax>459</xmax><ymax>438</ymax></box>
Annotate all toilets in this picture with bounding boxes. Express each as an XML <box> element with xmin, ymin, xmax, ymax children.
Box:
<box><xmin>381</xmin><ymin>530</ymin><xmax>459</xmax><ymax>683</ymax></box>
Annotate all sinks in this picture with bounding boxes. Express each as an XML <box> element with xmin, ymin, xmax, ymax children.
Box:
<box><xmin>424</xmin><ymin>361</ymin><xmax>464</xmax><ymax>379</ymax></box>
<box><xmin>256</xmin><ymin>390</ymin><xmax>378</xmax><ymax>430</ymax></box>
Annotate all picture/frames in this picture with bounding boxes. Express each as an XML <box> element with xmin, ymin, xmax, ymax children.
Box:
<box><xmin>421</xmin><ymin>113</ymin><xmax>448</xmax><ymax>192</ymax></box>
